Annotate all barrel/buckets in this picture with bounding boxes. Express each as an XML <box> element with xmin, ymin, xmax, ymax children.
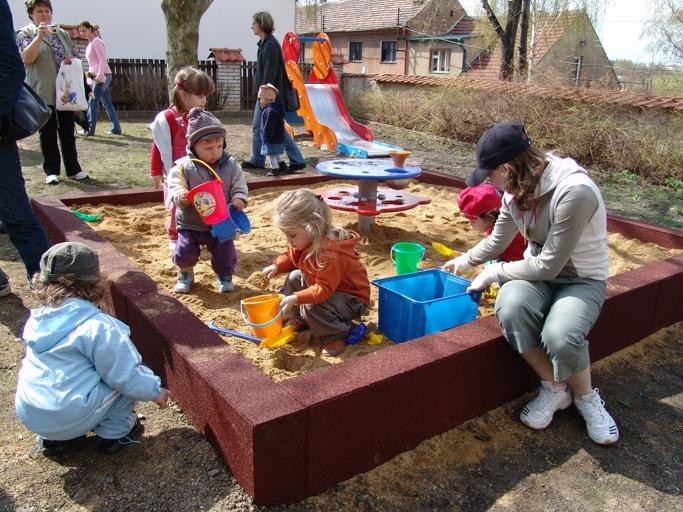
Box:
<box><xmin>179</xmin><ymin>158</ymin><xmax>229</xmax><ymax>226</ymax></box>
<box><xmin>389</xmin><ymin>241</ymin><xmax>427</xmax><ymax>275</ymax></box>
<box><xmin>209</xmin><ymin>204</ymin><xmax>251</xmax><ymax>244</ymax></box>
<box><xmin>240</xmin><ymin>293</ymin><xmax>286</xmax><ymax>339</ymax></box>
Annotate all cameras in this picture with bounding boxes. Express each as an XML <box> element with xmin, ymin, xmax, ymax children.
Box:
<box><xmin>46</xmin><ymin>24</ymin><xmax>59</xmax><ymax>35</ymax></box>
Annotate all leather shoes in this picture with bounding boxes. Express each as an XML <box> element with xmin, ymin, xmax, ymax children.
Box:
<box><xmin>284</xmin><ymin>163</ymin><xmax>306</xmax><ymax>174</ymax></box>
<box><xmin>241</xmin><ymin>160</ymin><xmax>266</xmax><ymax>169</ymax></box>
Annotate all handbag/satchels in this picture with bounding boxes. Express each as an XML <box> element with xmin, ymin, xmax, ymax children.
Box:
<box><xmin>285</xmin><ymin>86</ymin><xmax>300</xmax><ymax>112</ymax></box>
<box><xmin>55</xmin><ymin>58</ymin><xmax>88</xmax><ymax>113</ymax></box>
<box><xmin>0</xmin><ymin>81</ymin><xmax>54</xmax><ymax>143</ymax></box>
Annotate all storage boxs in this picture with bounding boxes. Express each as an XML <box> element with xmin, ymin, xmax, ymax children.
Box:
<box><xmin>370</xmin><ymin>268</ymin><xmax>486</xmax><ymax>345</ymax></box>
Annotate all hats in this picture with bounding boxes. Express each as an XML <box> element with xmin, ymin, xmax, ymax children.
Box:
<box><xmin>457</xmin><ymin>183</ymin><xmax>502</xmax><ymax>220</ymax></box>
<box><xmin>464</xmin><ymin>121</ymin><xmax>532</xmax><ymax>187</ymax></box>
<box><xmin>40</xmin><ymin>241</ymin><xmax>99</xmax><ymax>284</ymax></box>
<box><xmin>185</xmin><ymin>105</ymin><xmax>227</xmax><ymax>149</ymax></box>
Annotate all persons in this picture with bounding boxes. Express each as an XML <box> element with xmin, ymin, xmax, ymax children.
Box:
<box><xmin>148</xmin><ymin>65</ymin><xmax>215</xmax><ymax>252</ymax></box>
<box><xmin>239</xmin><ymin>10</ymin><xmax>307</xmax><ymax>173</ymax></box>
<box><xmin>441</xmin><ymin>121</ymin><xmax>619</xmax><ymax>445</ymax></box>
<box><xmin>257</xmin><ymin>83</ymin><xmax>287</xmax><ymax>175</ymax></box>
<box><xmin>164</xmin><ymin>106</ymin><xmax>249</xmax><ymax>294</ymax></box>
<box><xmin>456</xmin><ymin>183</ymin><xmax>529</xmax><ymax>261</ymax></box>
<box><xmin>0</xmin><ymin>0</ymin><xmax>48</xmax><ymax>297</ymax></box>
<box><xmin>13</xmin><ymin>240</ymin><xmax>171</xmax><ymax>456</ymax></box>
<box><xmin>74</xmin><ymin>21</ymin><xmax>122</xmax><ymax>135</ymax></box>
<box><xmin>14</xmin><ymin>1</ymin><xmax>90</xmax><ymax>185</ymax></box>
<box><xmin>261</xmin><ymin>189</ymin><xmax>370</xmax><ymax>357</ymax></box>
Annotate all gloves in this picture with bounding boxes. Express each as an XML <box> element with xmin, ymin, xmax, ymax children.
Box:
<box><xmin>466</xmin><ymin>263</ymin><xmax>501</xmax><ymax>294</ymax></box>
<box><xmin>440</xmin><ymin>254</ymin><xmax>472</xmax><ymax>277</ymax></box>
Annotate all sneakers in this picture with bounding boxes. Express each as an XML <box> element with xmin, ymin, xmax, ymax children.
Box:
<box><xmin>39</xmin><ymin>438</ymin><xmax>72</xmax><ymax>456</ymax></box>
<box><xmin>1</xmin><ymin>282</ymin><xmax>12</xmax><ymax>297</ymax></box>
<box><xmin>572</xmin><ymin>386</ymin><xmax>619</xmax><ymax>445</ymax></box>
<box><xmin>322</xmin><ymin>338</ymin><xmax>346</xmax><ymax>357</ymax></box>
<box><xmin>175</xmin><ymin>271</ymin><xmax>194</xmax><ymax>294</ymax></box>
<box><xmin>69</xmin><ymin>172</ymin><xmax>89</xmax><ymax>180</ymax></box>
<box><xmin>75</xmin><ymin>130</ymin><xmax>89</xmax><ymax>136</ymax></box>
<box><xmin>216</xmin><ymin>280</ymin><xmax>235</xmax><ymax>293</ymax></box>
<box><xmin>285</xmin><ymin>318</ymin><xmax>307</xmax><ymax>332</ymax></box>
<box><xmin>47</xmin><ymin>174</ymin><xmax>60</xmax><ymax>184</ymax></box>
<box><xmin>519</xmin><ymin>379</ymin><xmax>572</xmax><ymax>430</ymax></box>
<box><xmin>103</xmin><ymin>130</ymin><xmax>117</xmax><ymax>135</ymax></box>
<box><xmin>94</xmin><ymin>413</ymin><xmax>141</xmax><ymax>455</ymax></box>
